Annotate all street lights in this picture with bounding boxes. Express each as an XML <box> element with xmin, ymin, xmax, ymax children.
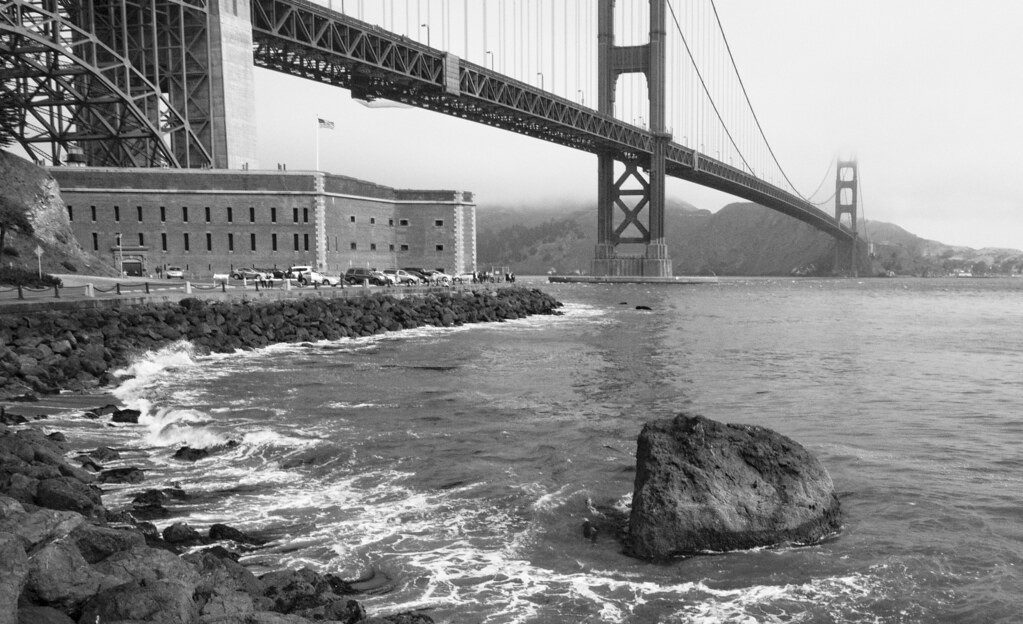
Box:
<box><xmin>717</xmin><ymin>151</ymin><xmax>720</xmax><ymax>161</ymax></box>
<box><xmin>741</xmin><ymin>163</ymin><xmax>764</xmax><ymax>180</ymax></box>
<box><xmin>578</xmin><ymin>90</ymin><xmax>583</xmax><ymax>106</ymax></box>
<box><xmin>421</xmin><ymin>24</ymin><xmax>429</xmax><ymax>46</ymax></box>
<box><xmin>537</xmin><ymin>73</ymin><xmax>543</xmax><ymax>90</ymax></box>
<box><xmin>639</xmin><ymin>116</ymin><xmax>644</xmax><ymax>123</ymax></box>
<box><xmin>684</xmin><ymin>136</ymin><xmax>688</xmax><ymax>147</ymax></box>
<box><xmin>487</xmin><ymin>51</ymin><xmax>493</xmax><ymax>71</ymax></box>
<box><xmin>729</xmin><ymin>157</ymin><xmax>733</xmax><ymax>166</ymax></box>
<box><xmin>701</xmin><ymin>144</ymin><xmax>705</xmax><ymax>154</ymax></box>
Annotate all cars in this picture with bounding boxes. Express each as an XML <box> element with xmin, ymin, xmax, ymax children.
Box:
<box><xmin>257</xmin><ymin>266</ymin><xmax>341</xmax><ymax>285</ymax></box>
<box><xmin>345</xmin><ymin>268</ymin><xmax>388</xmax><ymax>286</ymax></box>
<box><xmin>432</xmin><ymin>270</ymin><xmax>453</xmax><ymax>282</ymax></box>
<box><xmin>406</xmin><ymin>271</ymin><xmax>433</xmax><ymax>283</ymax></box>
<box><xmin>454</xmin><ymin>272</ymin><xmax>478</xmax><ymax>282</ymax></box>
<box><xmin>231</xmin><ymin>267</ymin><xmax>266</xmax><ymax>280</ymax></box>
<box><xmin>371</xmin><ymin>271</ymin><xmax>401</xmax><ymax>284</ymax></box>
<box><xmin>384</xmin><ymin>269</ymin><xmax>420</xmax><ymax>284</ymax></box>
<box><xmin>167</xmin><ymin>266</ymin><xmax>184</xmax><ymax>279</ymax></box>
<box><xmin>404</xmin><ymin>267</ymin><xmax>433</xmax><ymax>277</ymax></box>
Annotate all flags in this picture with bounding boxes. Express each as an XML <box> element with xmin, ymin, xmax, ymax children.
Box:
<box><xmin>318</xmin><ymin>118</ymin><xmax>334</xmax><ymax>130</ymax></box>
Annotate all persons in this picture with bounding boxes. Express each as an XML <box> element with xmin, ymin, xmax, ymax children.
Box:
<box><xmin>339</xmin><ymin>270</ymin><xmax>345</xmax><ymax>289</ymax></box>
<box><xmin>471</xmin><ymin>269</ymin><xmax>516</xmax><ymax>283</ymax></box>
<box><xmin>259</xmin><ymin>265</ymin><xmax>304</xmax><ymax>291</ymax></box>
<box><xmin>155</xmin><ymin>265</ymin><xmax>162</xmax><ymax>279</ymax></box>
